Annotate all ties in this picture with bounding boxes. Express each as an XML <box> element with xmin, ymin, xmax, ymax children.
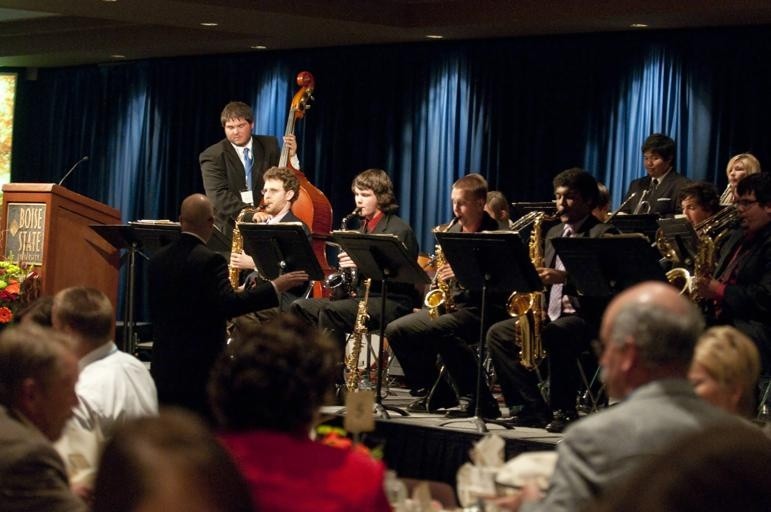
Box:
<box><xmin>709</xmin><ymin>238</ymin><xmax>757</xmax><ymax>305</ymax></box>
<box><xmin>360</xmin><ymin>219</ymin><xmax>368</xmax><ymax>232</ymax></box>
<box><xmin>242</xmin><ymin>147</ymin><xmax>254</xmax><ymax>192</ymax></box>
<box><xmin>547</xmin><ymin>227</ymin><xmax>570</xmax><ymax>321</ymax></box>
<box><xmin>647</xmin><ymin>176</ymin><xmax>661</xmax><ymax>196</ymax></box>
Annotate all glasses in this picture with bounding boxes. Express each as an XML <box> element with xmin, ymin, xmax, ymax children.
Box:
<box><xmin>261</xmin><ymin>187</ymin><xmax>290</xmax><ymax>194</ymax></box>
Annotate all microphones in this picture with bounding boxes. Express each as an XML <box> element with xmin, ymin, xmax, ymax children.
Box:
<box><xmin>57</xmin><ymin>155</ymin><xmax>90</xmax><ymax>185</ymax></box>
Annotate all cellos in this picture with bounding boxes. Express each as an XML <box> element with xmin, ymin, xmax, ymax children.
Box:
<box><xmin>260</xmin><ymin>72</ymin><xmax>334</xmax><ymax>302</ymax></box>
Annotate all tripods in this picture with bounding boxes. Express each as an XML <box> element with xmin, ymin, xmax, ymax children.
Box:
<box><xmin>439</xmin><ymin>275</ymin><xmax>515</xmax><ymax>433</ymax></box>
<box><xmin>336</xmin><ymin>280</ymin><xmax>409</xmax><ymax>421</ymax></box>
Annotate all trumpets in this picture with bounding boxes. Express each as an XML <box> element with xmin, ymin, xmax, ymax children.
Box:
<box><xmin>635</xmin><ymin>189</ymin><xmax>652</xmax><ymax>214</ymax></box>
<box><xmin>720</xmin><ymin>183</ymin><xmax>737</xmax><ymax>205</ymax></box>
<box><xmin>510</xmin><ymin>209</ymin><xmax>545</xmax><ymax>232</ymax></box>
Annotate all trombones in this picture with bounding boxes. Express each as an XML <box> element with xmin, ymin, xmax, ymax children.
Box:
<box><xmin>650</xmin><ymin>204</ymin><xmax>742</xmax><ymax>263</ymax></box>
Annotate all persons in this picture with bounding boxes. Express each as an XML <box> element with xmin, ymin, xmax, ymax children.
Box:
<box><xmin>52</xmin><ymin>287</ymin><xmax>158</xmax><ymax>511</ymax></box>
<box><xmin>145</xmin><ymin>195</ymin><xmax>309</xmax><ymax>425</ymax></box>
<box><xmin>385</xmin><ymin>174</ymin><xmax>510</xmax><ymax>418</ymax></box>
<box><xmin>695</xmin><ymin>173</ymin><xmax>771</xmax><ymax>379</ymax></box>
<box><xmin>496</xmin><ymin>283</ymin><xmax>771</xmax><ymax>512</ymax></box>
<box><xmin>680</xmin><ymin>184</ymin><xmax>719</xmax><ymax>227</ymax></box>
<box><xmin>93</xmin><ymin>412</ymin><xmax>253</xmax><ymax>511</ymax></box>
<box><xmin>0</xmin><ymin>325</ymin><xmax>95</xmax><ymax>512</ymax></box>
<box><xmin>230</xmin><ymin>167</ymin><xmax>313</xmax><ymax>334</ymax></box>
<box><xmin>484</xmin><ymin>191</ymin><xmax>513</xmax><ymax>228</ymax></box>
<box><xmin>209</xmin><ymin>314</ymin><xmax>393</xmax><ymax>512</ymax></box>
<box><xmin>199</xmin><ymin>101</ymin><xmax>301</xmax><ymax>264</ymax></box>
<box><xmin>14</xmin><ymin>298</ymin><xmax>52</xmax><ymax>327</ymax></box>
<box><xmin>486</xmin><ymin>170</ymin><xmax>621</xmax><ymax>432</ymax></box>
<box><xmin>289</xmin><ymin>170</ymin><xmax>419</xmax><ymax>386</ymax></box>
<box><xmin>592</xmin><ymin>182</ymin><xmax>611</xmax><ymax>223</ymax></box>
<box><xmin>618</xmin><ymin>134</ymin><xmax>691</xmax><ymax>215</ymax></box>
<box><xmin>688</xmin><ymin>327</ymin><xmax>760</xmax><ymax>416</ymax></box>
<box><xmin>719</xmin><ymin>154</ymin><xmax>761</xmax><ymax>205</ymax></box>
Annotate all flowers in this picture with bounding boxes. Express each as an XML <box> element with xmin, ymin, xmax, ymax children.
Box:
<box><xmin>316</xmin><ymin>426</ymin><xmax>385</xmax><ymax>461</ymax></box>
<box><xmin>0</xmin><ymin>250</ymin><xmax>40</xmax><ymax>326</ymax></box>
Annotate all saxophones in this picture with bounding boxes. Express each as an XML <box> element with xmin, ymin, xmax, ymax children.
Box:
<box><xmin>343</xmin><ymin>277</ymin><xmax>372</xmax><ymax>392</ymax></box>
<box><xmin>226</xmin><ymin>204</ymin><xmax>268</xmax><ymax>293</ymax></box>
<box><xmin>322</xmin><ymin>207</ymin><xmax>366</xmax><ymax>301</ymax></box>
<box><xmin>424</xmin><ymin>217</ymin><xmax>464</xmax><ymax>315</ymax></box>
<box><xmin>665</xmin><ymin>217</ymin><xmax>743</xmax><ymax>305</ymax></box>
<box><xmin>506</xmin><ymin>211</ymin><xmax>567</xmax><ymax>372</ymax></box>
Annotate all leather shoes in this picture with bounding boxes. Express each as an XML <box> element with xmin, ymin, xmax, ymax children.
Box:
<box><xmin>408</xmin><ymin>386</ymin><xmax>430</xmax><ymax>395</ymax></box>
<box><xmin>446</xmin><ymin>406</ymin><xmax>479</xmax><ymax>418</ymax></box>
<box><xmin>546</xmin><ymin>415</ymin><xmax>577</xmax><ymax>431</ymax></box>
<box><xmin>497</xmin><ymin>411</ymin><xmax>555</xmax><ymax>426</ymax></box>
<box><xmin>407</xmin><ymin>401</ymin><xmax>431</xmax><ymax>411</ymax></box>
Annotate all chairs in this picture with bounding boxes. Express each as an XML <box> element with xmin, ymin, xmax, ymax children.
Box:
<box><xmin>576</xmin><ymin>356</ymin><xmax>605</xmax><ymax>416</ymax></box>
<box><xmin>358</xmin><ymin>255</ymin><xmax>436</xmax><ymax>390</ymax></box>
<box><xmin>425</xmin><ymin>354</ymin><xmax>459</xmax><ymax>415</ymax></box>
<box><xmin>757</xmin><ymin>378</ymin><xmax>770</xmax><ymax>418</ymax></box>
<box><xmin>476</xmin><ymin>346</ymin><xmax>495</xmax><ymax>392</ymax></box>
